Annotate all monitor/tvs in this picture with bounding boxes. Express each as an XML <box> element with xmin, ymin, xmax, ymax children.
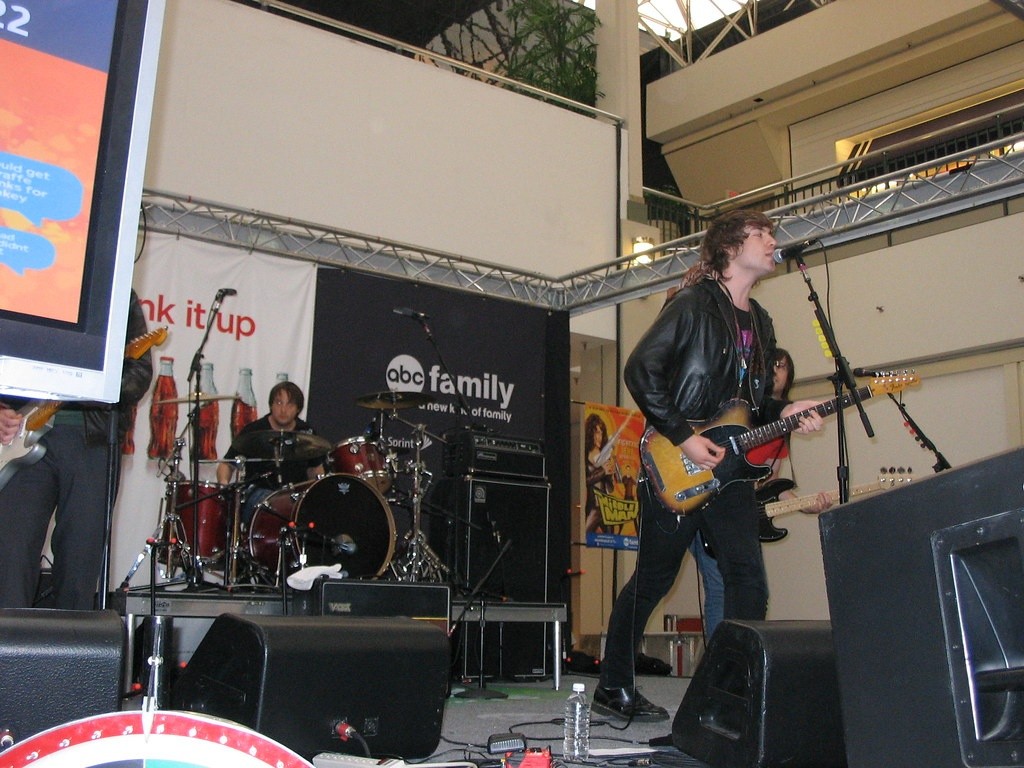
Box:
<box><xmin>1</xmin><ymin>0</ymin><xmax>168</xmax><ymax>406</ymax></box>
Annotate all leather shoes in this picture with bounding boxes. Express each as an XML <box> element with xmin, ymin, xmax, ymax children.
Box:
<box><xmin>591</xmin><ymin>685</ymin><xmax>670</xmax><ymax>723</ymax></box>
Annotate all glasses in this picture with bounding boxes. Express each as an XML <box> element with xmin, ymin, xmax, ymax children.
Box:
<box><xmin>774</xmin><ymin>360</ymin><xmax>785</xmax><ymax>369</ymax></box>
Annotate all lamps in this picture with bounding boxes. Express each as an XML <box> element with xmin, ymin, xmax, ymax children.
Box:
<box><xmin>632</xmin><ymin>236</ymin><xmax>657</xmax><ymax>262</ymax></box>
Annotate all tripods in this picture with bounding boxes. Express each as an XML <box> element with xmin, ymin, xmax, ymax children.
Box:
<box><xmin>117</xmin><ymin>400</ymin><xmax>283</xmax><ymax>597</ymax></box>
<box><xmin>383</xmin><ymin>400</ymin><xmax>450</xmax><ymax>583</ymax></box>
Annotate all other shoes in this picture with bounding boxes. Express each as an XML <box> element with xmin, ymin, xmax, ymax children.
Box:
<box><xmin>639</xmin><ymin>652</ymin><xmax>673</xmax><ymax>676</ymax></box>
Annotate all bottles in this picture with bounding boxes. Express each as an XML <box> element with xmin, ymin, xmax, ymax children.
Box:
<box><xmin>230</xmin><ymin>368</ymin><xmax>259</xmax><ymax>444</ymax></box>
<box><xmin>274</xmin><ymin>373</ymin><xmax>288</xmax><ymax>384</ymax></box>
<box><xmin>562</xmin><ymin>683</ymin><xmax>592</xmax><ymax>764</ymax></box>
<box><xmin>145</xmin><ymin>356</ymin><xmax>180</xmax><ymax>461</ymax></box>
<box><xmin>188</xmin><ymin>363</ymin><xmax>218</xmax><ymax>462</ymax></box>
<box><xmin>119</xmin><ymin>403</ymin><xmax>138</xmax><ymax>456</ymax></box>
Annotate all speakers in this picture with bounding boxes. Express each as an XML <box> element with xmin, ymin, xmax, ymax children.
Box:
<box><xmin>0</xmin><ymin>607</ymin><xmax>125</xmax><ymax>751</ymax></box>
<box><xmin>167</xmin><ymin>579</ymin><xmax>452</xmax><ymax>763</ymax></box>
<box><xmin>670</xmin><ymin>447</ymin><xmax>1024</xmax><ymax>767</ymax></box>
<box><xmin>430</xmin><ymin>475</ymin><xmax>551</xmax><ymax>605</ymax></box>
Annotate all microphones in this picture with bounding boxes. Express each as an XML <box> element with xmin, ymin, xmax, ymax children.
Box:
<box><xmin>393</xmin><ymin>306</ymin><xmax>430</xmax><ymax>319</ymax></box>
<box><xmin>340</xmin><ymin>542</ymin><xmax>357</xmax><ymax>555</ymax></box>
<box><xmin>271</xmin><ymin>446</ymin><xmax>283</xmax><ymax>484</ymax></box>
<box><xmin>363</xmin><ymin>413</ymin><xmax>381</xmax><ymax>437</ymax></box>
<box><xmin>773</xmin><ymin>237</ymin><xmax>818</xmax><ymax>264</ymax></box>
<box><xmin>218</xmin><ymin>288</ymin><xmax>238</xmax><ymax>295</ymax></box>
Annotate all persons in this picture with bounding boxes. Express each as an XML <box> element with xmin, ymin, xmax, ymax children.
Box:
<box><xmin>0</xmin><ymin>287</ymin><xmax>153</xmax><ymax>611</ymax></box>
<box><xmin>590</xmin><ymin>210</ymin><xmax>824</xmax><ymax>723</ymax></box>
<box><xmin>216</xmin><ymin>382</ymin><xmax>324</xmax><ymax>594</ymax></box>
<box><xmin>688</xmin><ymin>348</ymin><xmax>836</xmax><ymax>638</ymax></box>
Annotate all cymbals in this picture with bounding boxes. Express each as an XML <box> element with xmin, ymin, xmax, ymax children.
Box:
<box><xmin>353</xmin><ymin>389</ymin><xmax>432</xmax><ymax>410</ymax></box>
<box><xmin>230</xmin><ymin>430</ymin><xmax>337</xmax><ymax>462</ymax></box>
<box><xmin>155</xmin><ymin>392</ymin><xmax>242</xmax><ymax>405</ymax></box>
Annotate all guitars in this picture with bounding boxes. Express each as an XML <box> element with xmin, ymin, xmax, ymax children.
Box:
<box><xmin>637</xmin><ymin>367</ymin><xmax>922</xmax><ymax>515</ymax></box>
<box><xmin>753</xmin><ymin>465</ymin><xmax>917</xmax><ymax>542</ymax></box>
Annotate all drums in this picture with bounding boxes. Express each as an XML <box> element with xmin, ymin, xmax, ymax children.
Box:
<box><xmin>248</xmin><ymin>472</ymin><xmax>398</xmax><ymax>580</ymax></box>
<box><xmin>156</xmin><ymin>478</ymin><xmax>234</xmax><ymax>573</ymax></box>
<box><xmin>321</xmin><ymin>435</ymin><xmax>394</xmax><ymax>495</ymax></box>
